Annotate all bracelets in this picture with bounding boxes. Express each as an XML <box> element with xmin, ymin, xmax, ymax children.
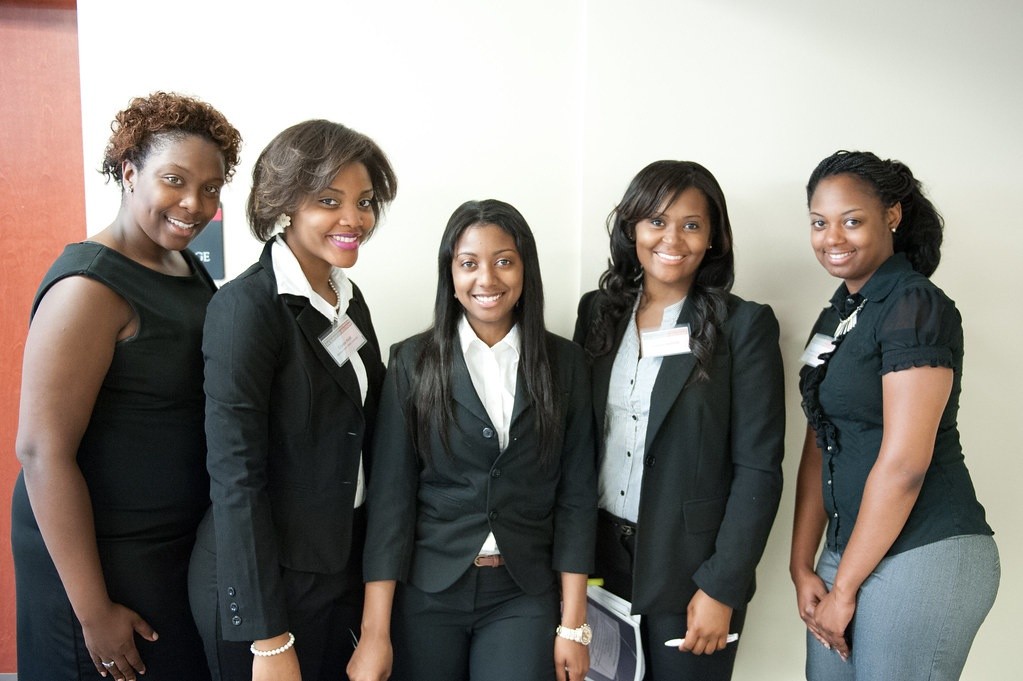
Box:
<box><xmin>250</xmin><ymin>633</ymin><xmax>294</xmax><ymax>656</ymax></box>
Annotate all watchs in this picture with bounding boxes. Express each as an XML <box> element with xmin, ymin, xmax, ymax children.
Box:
<box><xmin>557</xmin><ymin>623</ymin><xmax>593</xmax><ymax>645</ymax></box>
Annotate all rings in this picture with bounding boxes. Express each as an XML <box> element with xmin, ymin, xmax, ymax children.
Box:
<box><xmin>102</xmin><ymin>661</ymin><xmax>114</xmax><ymax>667</ymax></box>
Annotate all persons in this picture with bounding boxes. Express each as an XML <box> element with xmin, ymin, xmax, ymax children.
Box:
<box><xmin>790</xmin><ymin>149</ymin><xmax>1001</xmax><ymax>681</ymax></box>
<box><xmin>346</xmin><ymin>199</ymin><xmax>595</xmax><ymax>681</ymax></box>
<box><xmin>188</xmin><ymin>119</ymin><xmax>398</xmax><ymax>681</ymax></box>
<box><xmin>11</xmin><ymin>92</ymin><xmax>244</xmax><ymax>681</ymax></box>
<box><xmin>574</xmin><ymin>160</ymin><xmax>786</xmax><ymax>681</ymax></box>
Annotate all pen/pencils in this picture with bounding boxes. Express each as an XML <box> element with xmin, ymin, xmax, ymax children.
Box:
<box><xmin>665</xmin><ymin>633</ymin><xmax>739</xmax><ymax>647</ymax></box>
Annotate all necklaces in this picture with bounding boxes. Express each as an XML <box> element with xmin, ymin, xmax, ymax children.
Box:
<box><xmin>328</xmin><ymin>279</ymin><xmax>341</xmax><ymax>310</ymax></box>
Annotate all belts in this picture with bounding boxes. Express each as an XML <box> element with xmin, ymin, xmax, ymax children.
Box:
<box><xmin>598</xmin><ymin>508</ymin><xmax>636</xmax><ymax>535</ymax></box>
<box><xmin>472</xmin><ymin>555</ymin><xmax>504</xmax><ymax>568</ymax></box>
